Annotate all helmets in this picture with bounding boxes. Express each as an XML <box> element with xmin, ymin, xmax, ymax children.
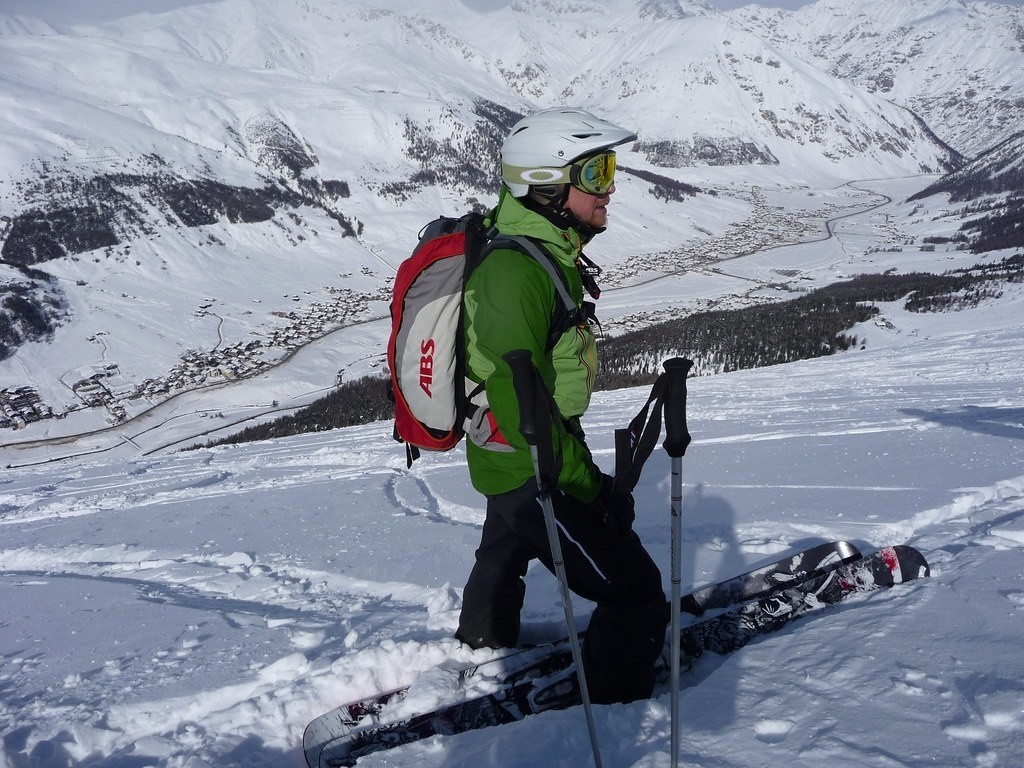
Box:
<box><xmin>501</xmin><ymin>108</ymin><xmax>637</xmax><ymax>197</ymax></box>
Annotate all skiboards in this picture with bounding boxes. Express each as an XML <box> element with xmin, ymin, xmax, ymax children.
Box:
<box><xmin>298</xmin><ymin>537</ymin><xmax>932</xmax><ymax>768</ymax></box>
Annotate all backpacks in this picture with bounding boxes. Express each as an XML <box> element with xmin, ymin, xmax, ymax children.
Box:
<box><xmin>388</xmin><ymin>212</ymin><xmax>577</xmax><ymax>469</ymax></box>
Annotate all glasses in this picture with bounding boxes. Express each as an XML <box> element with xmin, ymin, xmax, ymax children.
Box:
<box><xmin>502</xmin><ymin>150</ymin><xmax>617</xmax><ymax>194</ymax></box>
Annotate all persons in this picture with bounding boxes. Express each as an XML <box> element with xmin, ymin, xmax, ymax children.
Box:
<box><xmin>455</xmin><ymin>105</ymin><xmax>667</xmax><ymax>703</ymax></box>
